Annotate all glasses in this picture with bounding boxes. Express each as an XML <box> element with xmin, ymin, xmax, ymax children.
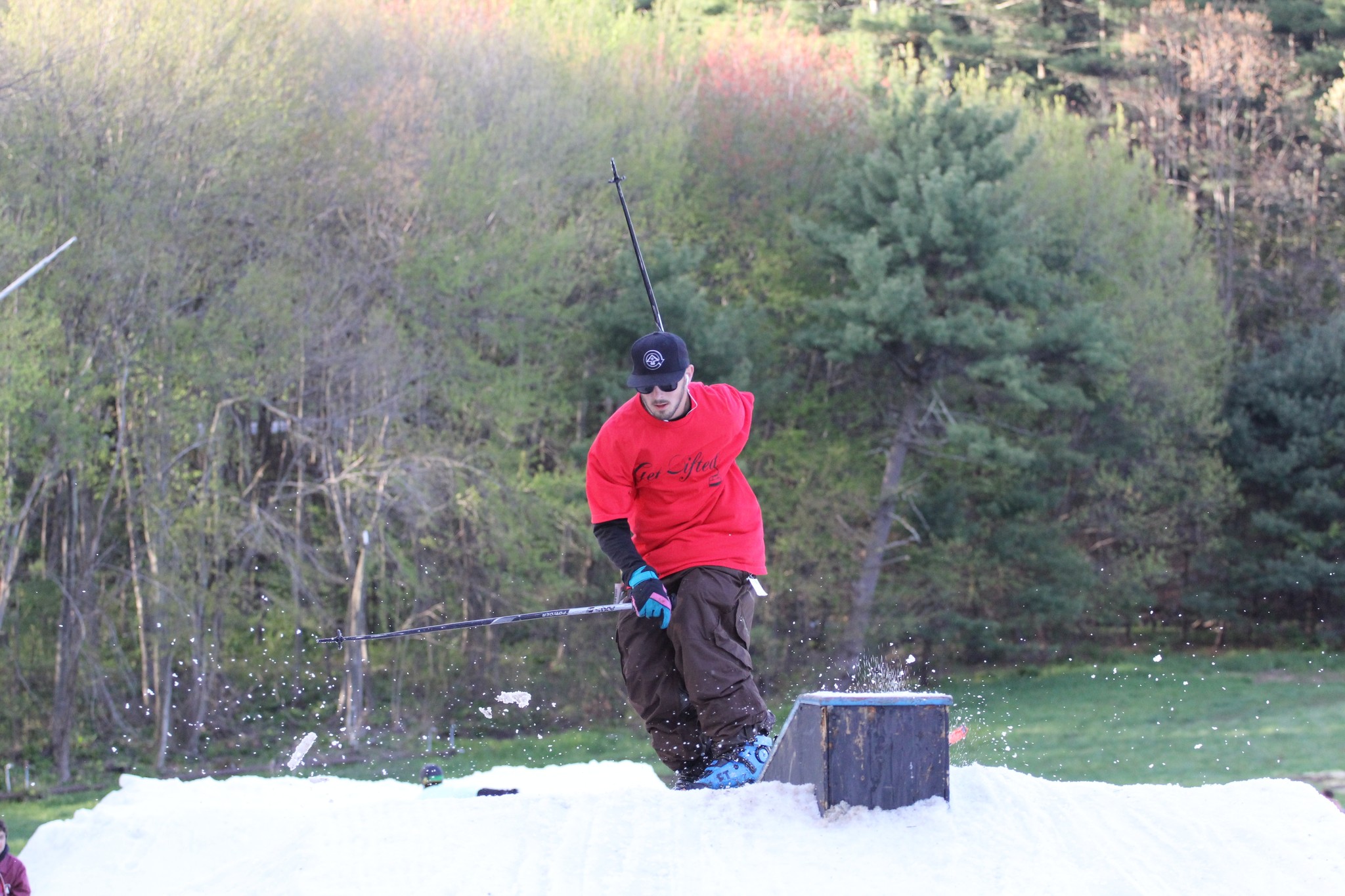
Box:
<box><xmin>635</xmin><ymin>383</ymin><xmax>677</xmax><ymax>394</ymax></box>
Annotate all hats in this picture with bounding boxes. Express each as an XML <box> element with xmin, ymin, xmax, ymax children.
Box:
<box><xmin>626</xmin><ymin>332</ymin><xmax>690</xmax><ymax>387</ymax></box>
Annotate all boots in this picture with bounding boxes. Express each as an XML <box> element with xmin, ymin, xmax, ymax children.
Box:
<box><xmin>670</xmin><ymin>773</ymin><xmax>696</xmax><ymax>790</ymax></box>
<box><xmin>692</xmin><ymin>735</ymin><xmax>772</xmax><ymax>789</ymax></box>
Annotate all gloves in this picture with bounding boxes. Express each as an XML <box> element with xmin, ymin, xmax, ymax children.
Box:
<box><xmin>629</xmin><ymin>565</ymin><xmax>671</xmax><ymax>629</ymax></box>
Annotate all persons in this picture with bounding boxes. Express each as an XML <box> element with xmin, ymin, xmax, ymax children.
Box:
<box><xmin>585</xmin><ymin>332</ymin><xmax>775</xmax><ymax>789</ymax></box>
<box><xmin>0</xmin><ymin>819</ymin><xmax>31</xmax><ymax>896</ymax></box>
